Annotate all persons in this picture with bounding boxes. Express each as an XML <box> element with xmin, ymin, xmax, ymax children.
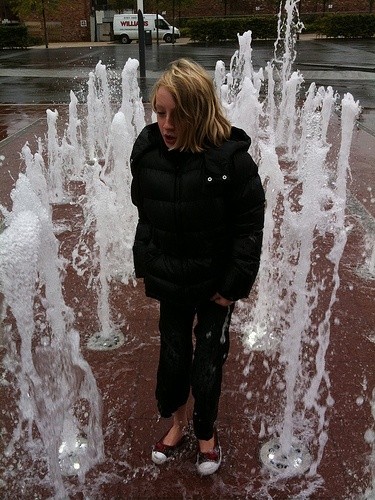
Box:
<box><xmin>128</xmin><ymin>58</ymin><xmax>266</xmax><ymax>476</ymax></box>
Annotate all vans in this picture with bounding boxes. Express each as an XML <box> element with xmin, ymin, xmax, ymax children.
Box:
<box><xmin>113</xmin><ymin>13</ymin><xmax>180</xmax><ymax>43</ymax></box>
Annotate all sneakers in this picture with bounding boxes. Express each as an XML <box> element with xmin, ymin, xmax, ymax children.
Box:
<box><xmin>151</xmin><ymin>424</ymin><xmax>187</xmax><ymax>464</ymax></box>
<box><xmin>195</xmin><ymin>425</ymin><xmax>222</xmax><ymax>476</ymax></box>
